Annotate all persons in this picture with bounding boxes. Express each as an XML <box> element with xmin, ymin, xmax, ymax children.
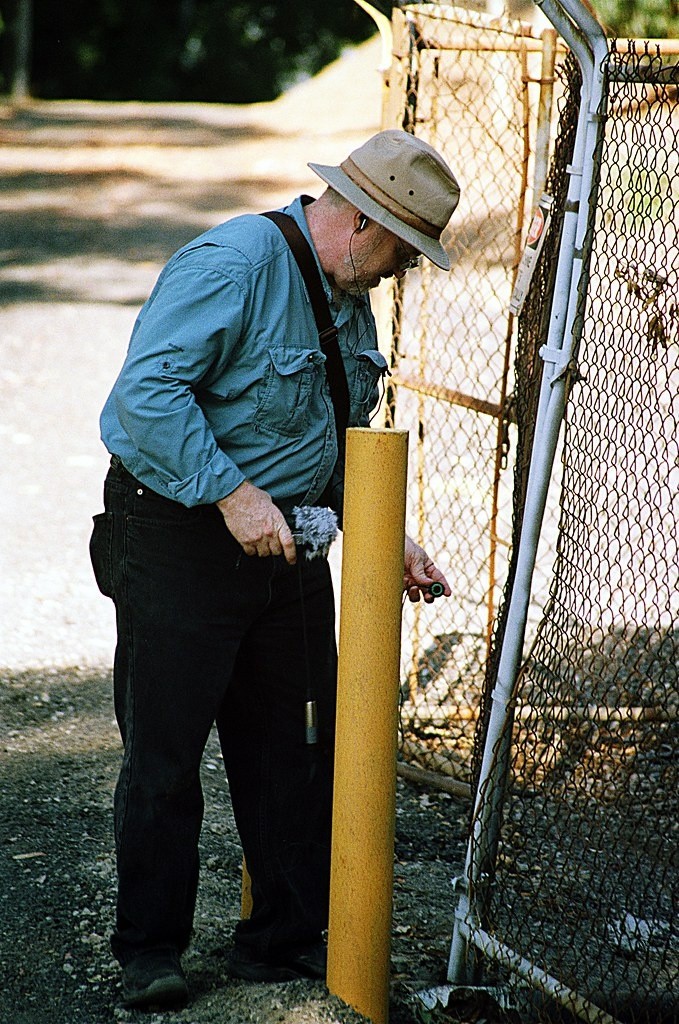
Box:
<box><xmin>89</xmin><ymin>129</ymin><xmax>460</xmax><ymax>1009</ymax></box>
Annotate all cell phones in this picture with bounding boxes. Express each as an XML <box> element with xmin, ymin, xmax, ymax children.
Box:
<box><xmin>286</xmin><ymin>513</ymin><xmax>307</xmax><ymax>544</ymax></box>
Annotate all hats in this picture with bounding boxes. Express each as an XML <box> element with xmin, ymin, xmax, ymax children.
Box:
<box><xmin>307</xmin><ymin>130</ymin><xmax>461</xmax><ymax>271</ymax></box>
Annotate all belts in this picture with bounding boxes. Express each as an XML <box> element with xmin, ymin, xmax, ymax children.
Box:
<box><xmin>110</xmin><ymin>454</ymin><xmax>131</xmax><ymax>475</ymax></box>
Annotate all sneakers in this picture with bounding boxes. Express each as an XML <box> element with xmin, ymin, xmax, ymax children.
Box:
<box><xmin>121</xmin><ymin>952</ymin><xmax>190</xmax><ymax>1009</ymax></box>
<box><xmin>231</xmin><ymin>945</ymin><xmax>326</xmax><ymax>981</ymax></box>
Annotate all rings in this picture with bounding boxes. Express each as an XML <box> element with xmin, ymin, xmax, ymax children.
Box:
<box><xmin>424</xmin><ymin>562</ymin><xmax>434</xmax><ymax>572</ymax></box>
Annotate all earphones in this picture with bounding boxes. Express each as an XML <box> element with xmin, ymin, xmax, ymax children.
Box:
<box><xmin>359</xmin><ymin>213</ymin><xmax>369</xmax><ymax>230</ymax></box>
<box><xmin>429</xmin><ymin>582</ymin><xmax>444</xmax><ymax>596</ymax></box>
